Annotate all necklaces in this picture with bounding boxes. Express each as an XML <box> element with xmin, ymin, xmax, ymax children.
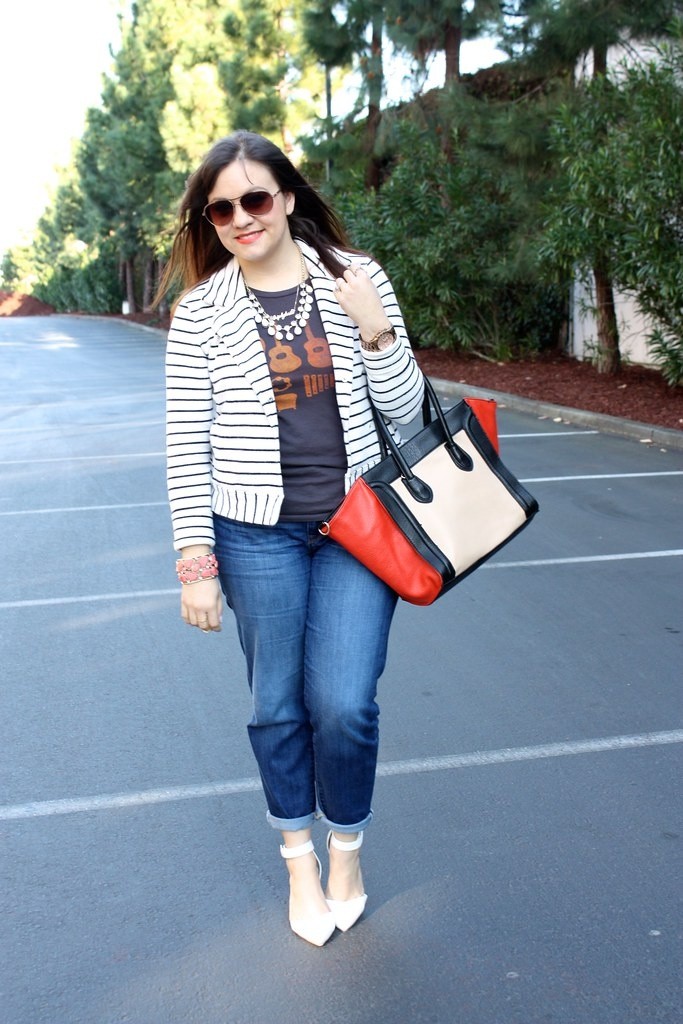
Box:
<box><xmin>242</xmin><ymin>241</ymin><xmax>314</xmax><ymax>342</ymax></box>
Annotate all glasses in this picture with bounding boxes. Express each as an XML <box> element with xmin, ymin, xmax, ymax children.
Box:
<box><xmin>202</xmin><ymin>188</ymin><xmax>285</xmax><ymax>227</ymax></box>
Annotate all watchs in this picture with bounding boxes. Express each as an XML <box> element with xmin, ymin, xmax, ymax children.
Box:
<box><xmin>358</xmin><ymin>321</ymin><xmax>398</xmax><ymax>353</ymax></box>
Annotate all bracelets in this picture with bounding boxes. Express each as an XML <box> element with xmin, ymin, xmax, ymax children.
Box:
<box><xmin>175</xmin><ymin>551</ymin><xmax>220</xmax><ymax>585</ymax></box>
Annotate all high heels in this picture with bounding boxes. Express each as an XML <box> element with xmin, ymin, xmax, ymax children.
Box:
<box><xmin>280</xmin><ymin>839</ymin><xmax>335</xmax><ymax>946</ymax></box>
<box><xmin>325</xmin><ymin>831</ymin><xmax>369</xmax><ymax>933</ymax></box>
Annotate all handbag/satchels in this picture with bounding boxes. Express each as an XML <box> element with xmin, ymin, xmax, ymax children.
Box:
<box><xmin>320</xmin><ymin>368</ymin><xmax>540</xmax><ymax>606</ymax></box>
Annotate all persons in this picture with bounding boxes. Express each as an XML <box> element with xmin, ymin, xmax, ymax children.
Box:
<box><xmin>164</xmin><ymin>130</ymin><xmax>423</xmax><ymax>949</ymax></box>
